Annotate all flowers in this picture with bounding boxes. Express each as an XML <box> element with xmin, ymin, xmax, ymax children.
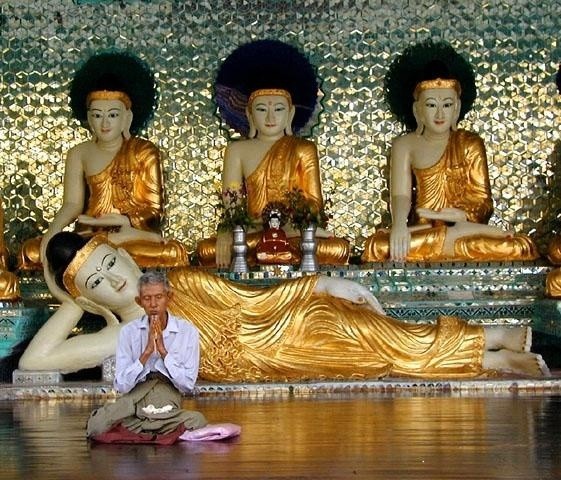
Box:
<box><xmin>212</xmin><ymin>182</ymin><xmax>254</xmax><ymax>231</ymax></box>
<box><xmin>282</xmin><ymin>186</ymin><xmax>335</xmax><ymax>231</ymax></box>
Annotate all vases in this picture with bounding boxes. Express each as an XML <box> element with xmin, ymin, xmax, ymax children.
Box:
<box><xmin>228</xmin><ymin>224</ymin><xmax>252</xmax><ymax>273</ymax></box>
<box><xmin>298</xmin><ymin>221</ymin><xmax>318</xmax><ymax>271</ymax></box>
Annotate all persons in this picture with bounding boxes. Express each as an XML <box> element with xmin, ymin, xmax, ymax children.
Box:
<box><xmin>85</xmin><ymin>271</ymin><xmax>208</xmax><ymax>441</ymax></box>
<box><xmin>18</xmin><ymin>231</ymin><xmax>552</xmax><ymax>383</ymax></box>
<box><xmin>362</xmin><ymin>39</ymin><xmax>540</xmax><ymax>261</ymax></box>
<box><xmin>19</xmin><ymin>53</ymin><xmax>188</xmax><ymax>268</ymax></box>
<box><xmin>193</xmin><ymin>40</ymin><xmax>351</xmax><ymax>266</ymax></box>
